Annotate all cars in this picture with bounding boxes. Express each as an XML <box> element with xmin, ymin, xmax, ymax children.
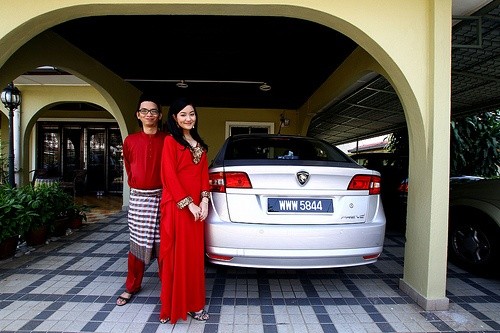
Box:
<box><xmin>204</xmin><ymin>133</ymin><xmax>386</xmax><ymax>269</ymax></box>
<box><xmin>350</xmin><ymin>152</ymin><xmax>500</xmax><ymax>273</ymax></box>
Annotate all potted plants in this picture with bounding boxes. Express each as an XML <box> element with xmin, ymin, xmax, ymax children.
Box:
<box><xmin>0</xmin><ymin>182</ymin><xmax>91</xmax><ymax>260</ymax></box>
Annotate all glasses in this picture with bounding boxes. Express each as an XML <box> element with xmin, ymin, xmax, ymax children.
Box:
<box><xmin>137</xmin><ymin>108</ymin><xmax>159</xmax><ymax>115</ymax></box>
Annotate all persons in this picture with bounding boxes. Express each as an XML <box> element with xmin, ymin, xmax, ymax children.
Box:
<box><xmin>117</xmin><ymin>96</ymin><xmax>167</xmax><ymax>306</ymax></box>
<box><xmin>159</xmin><ymin>98</ymin><xmax>211</xmax><ymax>323</ymax></box>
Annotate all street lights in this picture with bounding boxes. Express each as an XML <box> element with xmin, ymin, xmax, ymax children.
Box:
<box><xmin>0</xmin><ymin>81</ymin><xmax>22</xmax><ymax>189</ymax></box>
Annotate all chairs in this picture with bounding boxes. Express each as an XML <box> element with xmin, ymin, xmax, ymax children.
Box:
<box><xmin>62</xmin><ymin>170</ymin><xmax>85</xmax><ymax>199</ymax></box>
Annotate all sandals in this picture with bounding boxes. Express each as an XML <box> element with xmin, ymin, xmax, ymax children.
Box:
<box><xmin>116</xmin><ymin>290</ymin><xmax>134</xmax><ymax>306</ymax></box>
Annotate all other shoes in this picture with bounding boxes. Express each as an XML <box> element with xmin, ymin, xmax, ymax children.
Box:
<box><xmin>160</xmin><ymin>317</ymin><xmax>170</xmax><ymax>323</ymax></box>
<box><xmin>187</xmin><ymin>309</ymin><xmax>210</xmax><ymax>320</ymax></box>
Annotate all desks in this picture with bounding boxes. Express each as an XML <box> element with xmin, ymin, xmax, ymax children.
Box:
<box><xmin>33</xmin><ymin>178</ymin><xmax>66</xmax><ymax>194</ymax></box>
